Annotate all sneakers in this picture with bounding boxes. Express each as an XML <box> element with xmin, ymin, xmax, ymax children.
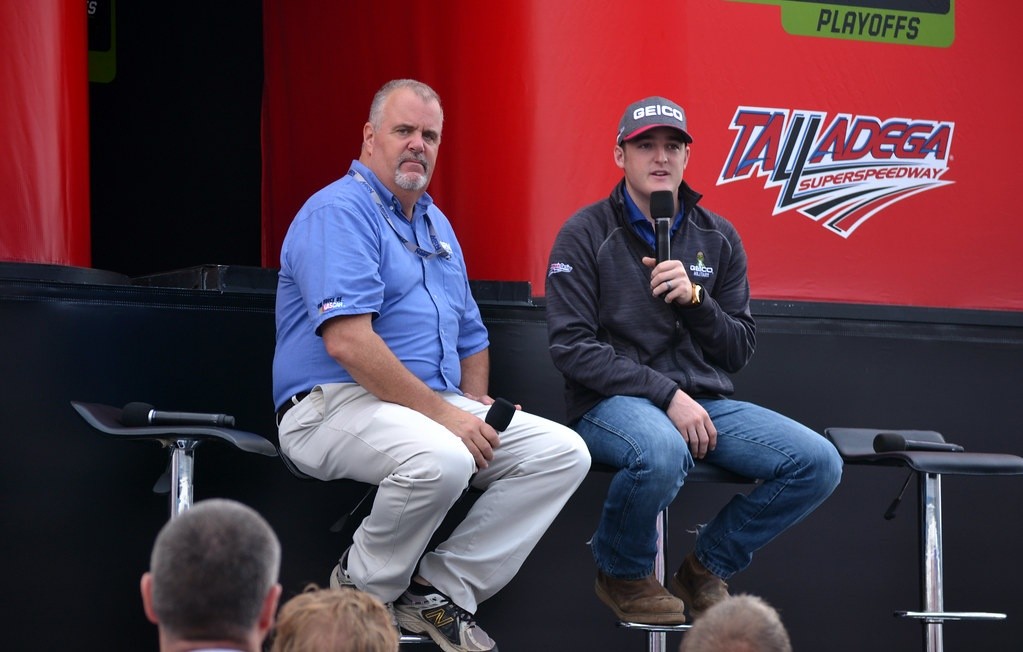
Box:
<box><xmin>391</xmin><ymin>576</ymin><xmax>497</xmax><ymax>652</ymax></box>
<box><xmin>330</xmin><ymin>545</ymin><xmax>401</xmax><ymax>637</ymax></box>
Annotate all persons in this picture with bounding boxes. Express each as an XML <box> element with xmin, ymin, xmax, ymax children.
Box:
<box><xmin>272</xmin><ymin>584</ymin><xmax>400</xmax><ymax>652</ymax></box>
<box><xmin>140</xmin><ymin>498</ymin><xmax>282</xmax><ymax>652</ymax></box>
<box><xmin>545</xmin><ymin>96</ymin><xmax>843</xmax><ymax>625</ymax></box>
<box><xmin>273</xmin><ymin>79</ymin><xmax>591</xmax><ymax>652</ymax></box>
<box><xmin>678</xmin><ymin>592</ymin><xmax>793</xmax><ymax>652</ymax></box>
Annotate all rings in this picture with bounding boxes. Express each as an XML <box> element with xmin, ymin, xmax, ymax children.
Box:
<box><xmin>666</xmin><ymin>281</ymin><xmax>672</xmax><ymax>290</ymax></box>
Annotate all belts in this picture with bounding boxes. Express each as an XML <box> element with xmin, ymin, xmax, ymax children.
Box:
<box><xmin>277</xmin><ymin>391</ymin><xmax>310</xmax><ymax>425</ymax></box>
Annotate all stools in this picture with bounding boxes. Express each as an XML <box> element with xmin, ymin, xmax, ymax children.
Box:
<box><xmin>73</xmin><ymin>401</ymin><xmax>277</xmax><ymax>523</ymax></box>
<box><xmin>621</xmin><ymin>462</ymin><xmax>762</xmax><ymax>652</ymax></box>
<box><xmin>826</xmin><ymin>428</ymin><xmax>1023</xmax><ymax>652</ymax></box>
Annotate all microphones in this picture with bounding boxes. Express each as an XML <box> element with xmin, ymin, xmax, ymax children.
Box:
<box><xmin>650</xmin><ymin>191</ymin><xmax>675</xmax><ymax>297</ymax></box>
<box><xmin>873</xmin><ymin>432</ymin><xmax>964</xmax><ymax>453</ymax></box>
<box><xmin>463</xmin><ymin>398</ymin><xmax>516</xmax><ymax>492</ymax></box>
<box><xmin>121</xmin><ymin>401</ymin><xmax>235</xmax><ymax>429</ymax></box>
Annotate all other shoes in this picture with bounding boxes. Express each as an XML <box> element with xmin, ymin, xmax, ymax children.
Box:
<box><xmin>594</xmin><ymin>566</ymin><xmax>687</xmax><ymax>625</ymax></box>
<box><xmin>672</xmin><ymin>552</ymin><xmax>734</xmax><ymax>621</ymax></box>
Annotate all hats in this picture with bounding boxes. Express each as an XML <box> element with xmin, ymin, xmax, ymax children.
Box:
<box><xmin>617</xmin><ymin>95</ymin><xmax>692</xmax><ymax>147</ymax></box>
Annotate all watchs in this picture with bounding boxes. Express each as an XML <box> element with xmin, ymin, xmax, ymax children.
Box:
<box><xmin>690</xmin><ymin>283</ymin><xmax>702</xmax><ymax>305</ymax></box>
